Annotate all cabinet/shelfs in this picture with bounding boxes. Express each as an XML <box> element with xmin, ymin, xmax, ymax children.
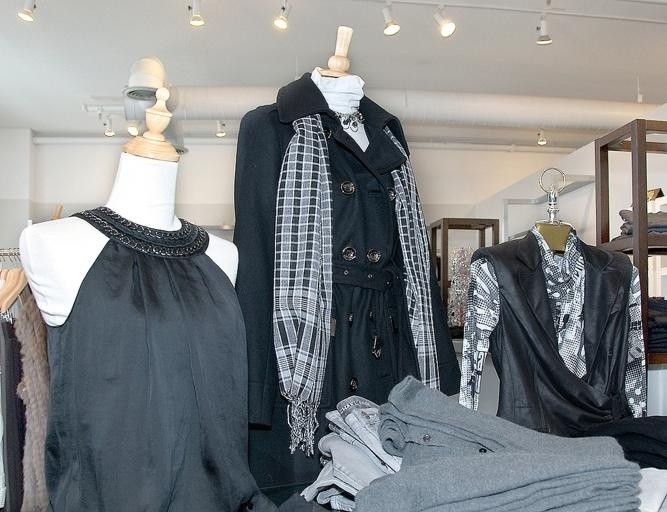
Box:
<box><xmin>591</xmin><ymin>117</ymin><xmax>667</xmax><ymax>364</ymax></box>
<box><xmin>425</xmin><ymin>218</ymin><xmax>498</xmax><ymax>338</ymax></box>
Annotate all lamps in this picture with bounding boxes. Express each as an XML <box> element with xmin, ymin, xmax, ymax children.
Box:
<box><xmin>188</xmin><ymin>1</ymin><xmax>206</xmax><ymax>25</ymax></box>
<box><xmin>102</xmin><ymin>116</ymin><xmax>114</xmax><ymax>136</ymax></box>
<box><xmin>535</xmin><ymin>128</ymin><xmax>547</xmax><ymax>145</ymax></box>
<box><xmin>431</xmin><ymin>3</ymin><xmax>457</xmax><ymax>39</ymax></box>
<box><xmin>125</xmin><ymin>116</ymin><xmax>136</xmax><ymax>136</ymax></box>
<box><xmin>535</xmin><ymin>32</ymin><xmax>554</xmax><ymax>44</ymax></box>
<box><xmin>272</xmin><ymin>1</ymin><xmax>291</xmax><ymax>32</ymax></box>
<box><xmin>214</xmin><ymin>117</ymin><xmax>225</xmax><ymax>139</ymax></box>
<box><xmin>381</xmin><ymin>1</ymin><xmax>401</xmax><ymax>38</ymax></box>
<box><xmin>16</xmin><ymin>2</ymin><xmax>34</xmax><ymax>20</ymax></box>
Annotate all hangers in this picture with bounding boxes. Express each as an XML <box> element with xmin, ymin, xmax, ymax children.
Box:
<box><xmin>533</xmin><ymin>168</ymin><xmax>571</xmax><ymax>252</ymax></box>
<box><xmin>0</xmin><ymin>246</ymin><xmax>28</xmax><ymax>316</ymax></box>
